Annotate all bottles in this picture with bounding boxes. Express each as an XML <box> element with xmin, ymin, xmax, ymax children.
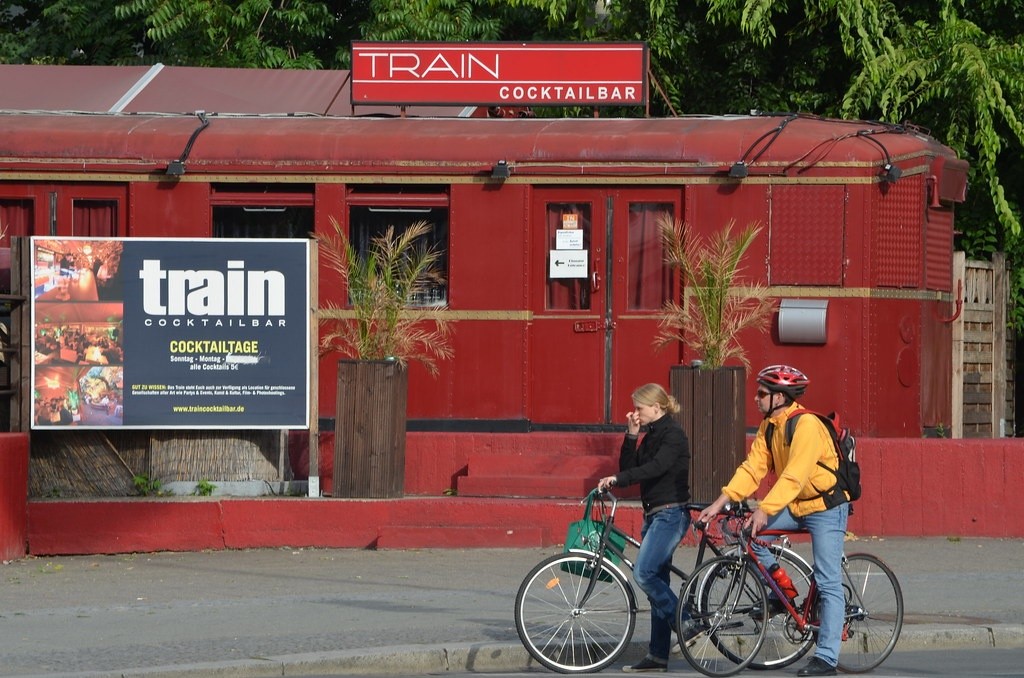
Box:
<box><xmin>770</xmin><ymin>563</ymin><xmax>799</xmax><ymax>600</ymax></box>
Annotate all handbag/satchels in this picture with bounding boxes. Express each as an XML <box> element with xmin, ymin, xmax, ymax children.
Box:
<box><xmin>559</xmin><ymin>490</ymin><xmax>627</xmax><ymax>584</ymax></box>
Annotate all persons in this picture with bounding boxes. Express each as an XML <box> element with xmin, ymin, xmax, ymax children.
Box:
<box><xmin>700</xmin><ymin>364</ymin><xmax>853</xmax><ymax>676</ymax></box>
<box><xmin>598</xmin><ymin>383</ymin><xmax>708</xmax><ymax>672</ymax></box>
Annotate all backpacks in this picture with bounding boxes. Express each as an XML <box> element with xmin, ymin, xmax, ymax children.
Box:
<box><xmin>765</xmin><ymin>409</ymin><xmax>862</xmax><ymax>503</ymax></box>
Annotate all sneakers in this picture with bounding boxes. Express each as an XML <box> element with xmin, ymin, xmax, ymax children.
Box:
<box><xmin>748</xmin><ymin>590</ymin><xmax>797</xmax><ymax>620</ymax></box>
<box><xmin>622</xmin><ymin>656</ymin><xmax>668</xmax><ymax>674</ymax></box>
<box><xmin>671</xmin><ymin>623</ymin><xmax>707</xmax><ymax>655</ymax></box>
<box><xmin>797</xmin><ymin>656</ymin><xmax>837</xmax><ymax>677</ymax></box>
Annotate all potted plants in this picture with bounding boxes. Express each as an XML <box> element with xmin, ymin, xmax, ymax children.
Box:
<box><xmin>305</xmin><ymin>212</ymin><xmax>458</xmax><ymax>500</ymax></box>
<box><xmin>651</xmin><ymin>211</ymin><xmax>781</xmax><ymax>505</ymax></box>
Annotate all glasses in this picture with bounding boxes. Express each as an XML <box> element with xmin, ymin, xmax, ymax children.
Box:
<box><xmin>757</xmin><ymin>389</ymin><xmax>777</xmax><ymax>399</ymax></box>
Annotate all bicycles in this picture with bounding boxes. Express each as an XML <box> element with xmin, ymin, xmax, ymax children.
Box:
<box><xmin>676</xmin><ymin>517</ymin><xmax>906</xmax><ymax>678</ymax></box>
<box><xmin>513</xmin><ymin>479</ymin><xmax>814</xmax><ymax>674</ymax></box>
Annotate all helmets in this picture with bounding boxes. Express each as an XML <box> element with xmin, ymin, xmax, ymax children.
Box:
<box><xmin>755</xmin><ymin>363</ymin><xmax>810</xmax><ymax>402</ymax></box>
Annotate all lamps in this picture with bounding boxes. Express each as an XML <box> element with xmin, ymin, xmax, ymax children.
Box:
<box><xmin>883</xmin><ymin>164</ymin><xmax>902</xmax><ymax>183</ymax></box>
<box><xmin>728</xmin><ymin>161</ymin><xmax>747</xmax><ymax>178</ymax></box>
<box><xmin>491</xmin><ymin>160</ymin><xmax>510</xmax><ymax>179</ymax></box>
<box><xmin>165</xmin><ymin>159</ymin><xmax>185</xmax><ymax>176</ymax></box>
